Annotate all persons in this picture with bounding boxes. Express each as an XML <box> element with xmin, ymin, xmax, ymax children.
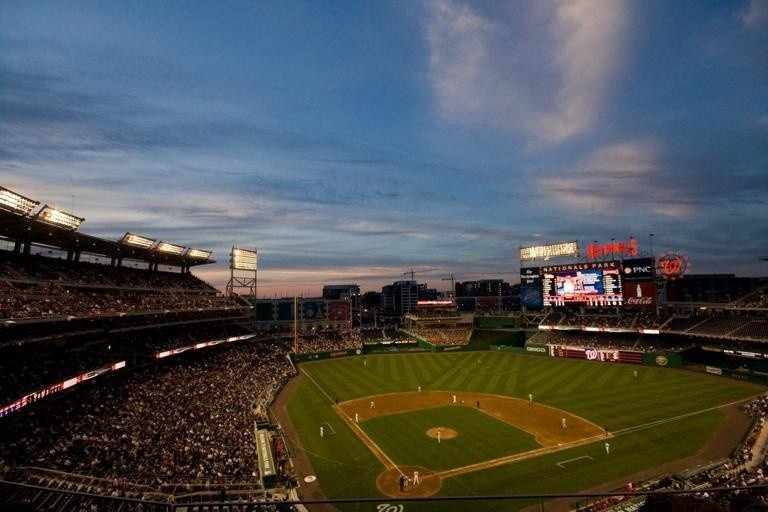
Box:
<box><xmin>474</xmin><ymin>305</ymin><xmax>767</xmax><ymax>378</ymax></box>
<box><xmin>398</xmin><ymin>470</ymin><xmax>421</xmax><ymax>493</ymax></box>
<box><xmin>1</xmin><ymin>249</ymin><xmax>301</xmax><ymax>511</ymax></box>
<box><xmin>288</xmin><ymin>307</ymin><xmax>470</xmax><ymax>350</ymax></box>
<box><xmin>418</xmin><ymin>384</ymin><xmax>481</xmax><ymax>443</ymax></box>
<box><xmin>527</xmin><ymin>393</ymin><xmax>568</xmax><ymax>429</ymax></box>
<box><xmin>576</xmin><ymin>389</ymin><xmax>765</xmax><ymax>510</ymax></box>
<box><xmin>318</xmin><ymin>358</ymin><xmax>376</xmax><ymax>438</ymax></box>
